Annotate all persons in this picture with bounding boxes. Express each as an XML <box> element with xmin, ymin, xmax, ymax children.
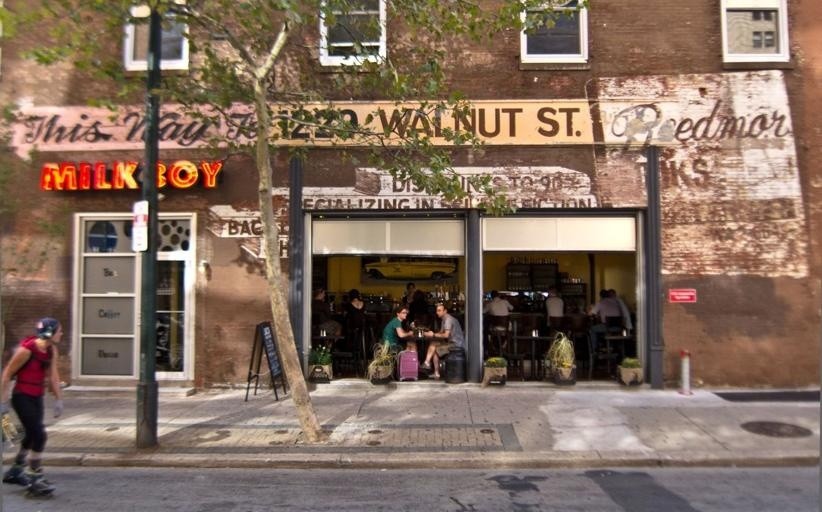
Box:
<box><xmin>537</xmin><ymin>288</ymin><xmax>564</xmax><ymax>345</ymax></box>
<box><xmin>313</xmin><ymin>288</ymin><xmax>331</xmax><ymax>336</ymax></box>
<box><xmin>484</xmin><ymin>290</ymin><xmax>515</xmax><ymax>351</ymax></box>
<box><xmin>534</xmin><ymin>294</ymin><xmax>546</xmax><ymax>312</ymax></box>
<box><xmin>419</xmin><ymin>304</ymin><xmax>465</xmax><ymax>379</ymax></box>
<box><xmin>409</xmin><ymin>289</ymin><xmax>431</xmax><ymax>329</ymax></box>
<box><xmin>343</xmin><ymin>289</ymin><xmax>366</xmax><ymax>350</ymax></box>
<box><xmin>1</xmin><ymin>316</ymin><xmax>64</xmax><ymax>495</ymax></box>
<box><xmin>403</xmin><ymin>283</ymin><xmax>416</xmax><ymax>306</ymax></box>
<box><xmin>586</xmin><ymin>289</ymin><xmax>621</xmax><ymax>356</ymax></box>
<box><xmin>607</xmin><ymin>289</ymin><xmax>634</xmax><ymax>349</ymax></box>
<box><xmin>382</xmin><ymin>306</ymin><xmax>414</xmax><ymax>350</ymax></box>
<box><xmin>516</xmin><ymin>290</ymin><xmax>531</xmax><ymax>304</ymax></box>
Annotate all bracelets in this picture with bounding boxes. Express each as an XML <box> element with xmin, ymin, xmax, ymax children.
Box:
<box><xmin>433</xmin><ymin>334</ymin><xmax>436</xmax><ymax>340</ymax></box>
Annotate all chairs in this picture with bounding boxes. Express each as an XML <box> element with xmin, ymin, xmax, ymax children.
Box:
<box><xmin>585</xmin><ymin>315</ymin><xmax>624</xmax><ymax>381</ymax></box>
<box><xmin>480</xmin><ymin>314</ymin><xmax>526</xmax><ymax>382</ymax></box>
<box><xmin>549</xmin><ymin>316</ymin><xmax>573</xmax><ymax>332</ymax></box>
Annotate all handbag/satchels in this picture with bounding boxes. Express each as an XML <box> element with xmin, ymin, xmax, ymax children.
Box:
<box><xmin>308</xmin><ymin>366</ymin><xmax>330</xmax><ymax>381</ymax></box>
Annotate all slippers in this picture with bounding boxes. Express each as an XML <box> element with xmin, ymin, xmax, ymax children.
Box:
<box><xmin>428</xmin><ymin>375</ymin><xmax>441</xmax><ymax>380</ymax></box>
<box><xmin>420</xmin><ymin>363</ymin><xmax>431</xmax><ymax>369</ymax></box>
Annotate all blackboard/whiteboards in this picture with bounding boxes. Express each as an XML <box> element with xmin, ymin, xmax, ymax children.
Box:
<box><xmin>248</xmin><ymin>322</ymin><xmax>282</xmax><ymax>381</ymax></box>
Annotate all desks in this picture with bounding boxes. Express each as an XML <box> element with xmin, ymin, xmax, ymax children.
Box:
<box><xmin>603</xmin><ymin>334</ymin><xmax>633</xmax><ymax>380</ymax></box>
<box><xmin>510</xmin><ymin>336</ymin><xmax>555</xmax><ymax>381</ymax></box>
<box><xmin>405</xmin><ymin>335</ymin><xmax>444</xmax><ymax>379</ymax></box>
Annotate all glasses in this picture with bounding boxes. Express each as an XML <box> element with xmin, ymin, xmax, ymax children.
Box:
<box><xmin>400</xmin><ymin>312</ymin><xmax>408</xmax><ymax>314</ymax></box>
<box><xmin>437</xmin><ymin>309</ymin><xmax>444</xmax><ymax>311</ymax></box>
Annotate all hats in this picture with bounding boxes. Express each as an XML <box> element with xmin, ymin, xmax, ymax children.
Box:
<box><xmin>35</xmin><ymin>317</ymin><xmax>59</xmax><ymax>339</ymax></box>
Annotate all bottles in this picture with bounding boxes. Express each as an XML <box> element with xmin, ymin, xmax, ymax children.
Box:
<box><xmin>622</xmin><ymin>330</ymin><xmax>629</xmax><ymax>336</ymax></box>
<box><xmin>531</xmin><ymin>329</ymin><xmax>538</xmax><ymax>338</ymax></box>
<box><xmin>320</xmin><ymin>330</ymin><xmax>326</xmax><ymax>337</ymax></box>
<box><xmin>419</xmin><ymin>330</ymin><xmax>424</xmax><ymax>337</ymax></box>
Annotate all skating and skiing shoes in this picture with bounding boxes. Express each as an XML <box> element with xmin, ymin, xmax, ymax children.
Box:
<box><xmin>21</xmin><ymin>468</ymin><xmax>56</xmax><ymax>500</ymax></box>
<box><xmin>2</xmin><ymin>463</ymin><xmax>32</xmax><ymax>485</ymax></box>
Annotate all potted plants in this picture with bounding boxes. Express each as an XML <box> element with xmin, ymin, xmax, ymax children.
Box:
<box><xmin>618</xmin><ymin>356</ymin><xmax>642</xmax><ymax>384</ymax></box>
<box><xmin>548</xmin><ymin>330</ymin><xmax>576</xmax><ymax>380</ymax></box>
<box><xmin>482</xmin><ymin>358</ymin><xmax>507</xmax><ymax>384</ymax></box>
<box><xmin>308</xmin><ymin>342</ymin><xmax>336</xmax><ymax>384</ymax></box>
<box><xmin>367</xmin><ymin>337</ymin><xmax>402</xmax><ymax>384</ymax></box>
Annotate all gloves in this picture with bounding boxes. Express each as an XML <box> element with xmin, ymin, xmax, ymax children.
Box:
<box><xmin>1</xmin><ymin>402</ymin><xmax>9</xmax><ymax>415</ymax></box>
<box><xmin>54</xmin><ymin>400</ymin><xmax>63</xmax><ymax>418</ymax></box>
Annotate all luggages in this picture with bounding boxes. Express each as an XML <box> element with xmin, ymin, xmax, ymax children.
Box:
<box><xmin>396</xmin><ymin>351</ymin><xmax>419</xmax><ymax>381</ymax></box>
